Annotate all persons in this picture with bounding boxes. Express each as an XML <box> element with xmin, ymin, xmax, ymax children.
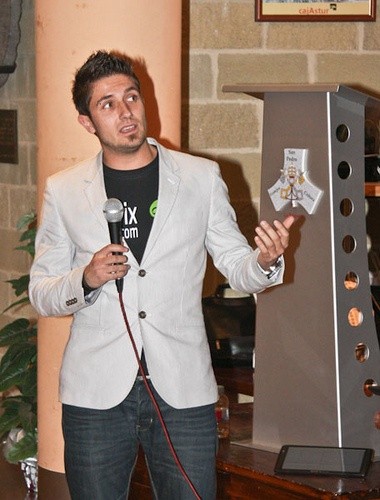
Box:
<box><xmin>29</xmin><ymin>51</ymin><xmax>296</xmax><ymax>500</ymax></box>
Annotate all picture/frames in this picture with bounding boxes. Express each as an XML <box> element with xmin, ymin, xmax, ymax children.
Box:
<box><xmin>256</xmin><ymin>0</ymin><xmax>376</xmax><ymax>22</ymax></box>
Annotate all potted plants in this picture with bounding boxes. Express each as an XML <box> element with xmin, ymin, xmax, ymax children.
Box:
<box><xmin>1</xmin><ymin>210</ymin><xmax>41</xmax><ymax>500</ymax></box>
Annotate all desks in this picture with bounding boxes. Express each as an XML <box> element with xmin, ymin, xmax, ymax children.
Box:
<box><xmin>139</xmin><ymin>363</ymin><xmax>380</xmax><ymax>500</ymax></box>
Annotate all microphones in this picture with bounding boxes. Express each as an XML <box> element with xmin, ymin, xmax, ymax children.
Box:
<box><xmin>103</xmin><ymin>198</ymin><xmax>124</xmax><ymax>292</ymax></box>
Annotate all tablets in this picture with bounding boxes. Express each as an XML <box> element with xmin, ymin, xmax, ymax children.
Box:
<box><xmin>274</xmin><ymin>445</ymin><xmax>374</xmax><ymax>477</ymax></box>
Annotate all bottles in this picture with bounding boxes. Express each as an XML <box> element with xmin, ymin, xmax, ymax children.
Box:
<box><xmin>214</xmin><ymin>385</ymin><xmax>231</xmax><ymax>439</ymax></box>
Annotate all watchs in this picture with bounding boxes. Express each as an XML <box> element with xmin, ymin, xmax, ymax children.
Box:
<box><xmin>258</xmin><ymin>259</ymin><xmax>280</xmax><ymax>279</ymax></box>
